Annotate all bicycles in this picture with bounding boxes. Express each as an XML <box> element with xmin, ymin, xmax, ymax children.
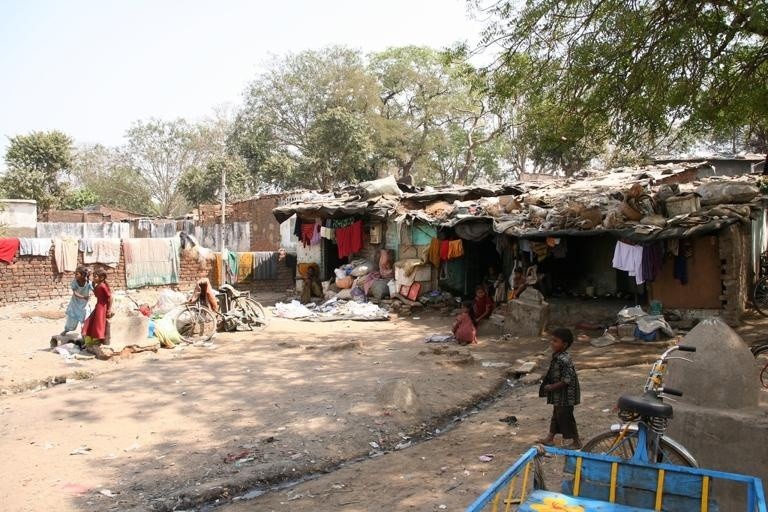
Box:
<box><xmin>750</xmin><ymin>250</ymin><xmax>768</xmax><ymax>388</ymax></box>
<box><xmin>174</xmin><ymin>283</ymin><xmax>267</xmax><ymax>344</ymax></box>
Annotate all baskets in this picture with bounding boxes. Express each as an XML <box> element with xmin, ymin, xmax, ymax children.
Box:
<box><xmin>623</xmin><ymin>197</ymin><xmax>647</xmax><ymax>220</ymax></box>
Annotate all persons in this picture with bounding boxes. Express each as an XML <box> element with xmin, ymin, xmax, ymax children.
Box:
<box><xmin>484</xmin><ymin>264</ymin><xmax>497</xmax><ymax>294</ymax></box>
<box><xmin>511</xmin><ymin>267</ymin><xmax>529</xmax><ymax>299</ymax></box>
<box><xmin>472</xmin><ymin>285</ymin><xmax>495</xmax><ymax>325</ymax></box>
<box><xmin>62</xmin><ymin>268</ymin><xmax>94</xmax><ymax>336</ymax></box>
<box><xmin>493</xmin><ymin>272</ymin><xmax>510</xmax><ymax>304</ymax></box>
<box><xmin>82</xmin><ymin>269</ymin><xmax>112</xmax><ymax>345</ymax></box>
<box><xmin>536</xmin><ymin>328</ymin><xmax>583</xmax><ymax>451</ymax></box>
<box><xmin>452</xmin><ymin>301</ymin><xmax>478</xmax><ymax>345</ymax></box>
<box><xmin>298</xmin><ymin>262</ymin><xmax>323</xmax><ymax>300</ymax></box>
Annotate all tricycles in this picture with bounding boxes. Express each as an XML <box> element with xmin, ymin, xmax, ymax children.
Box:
<box><xmin>467</xmin><ymin>346</ymin><xmax>767</xmax><ymax>512</ymax></box>
